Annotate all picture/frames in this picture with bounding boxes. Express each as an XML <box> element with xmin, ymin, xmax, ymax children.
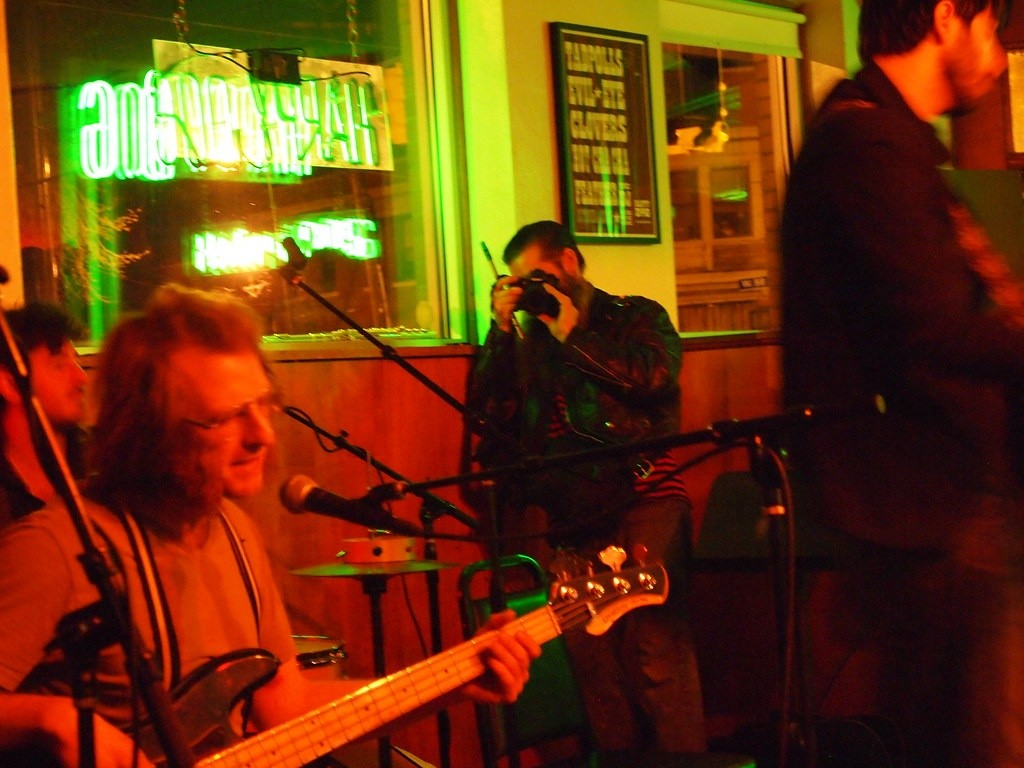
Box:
<box><xmin>548</xmin><ymin>20</ymin><xmax>664</xmax><ymax>245</ymax></box>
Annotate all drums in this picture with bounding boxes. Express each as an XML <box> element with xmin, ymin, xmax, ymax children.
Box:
<box><xmin>335</xmin><ymin>534</ymin><xmax>419</xmax><ymax>565</ymax></box>
<box><xmin>289</xmin><ymin>635</ymin><xmax>352</xmax><ymax>681</ymax></box>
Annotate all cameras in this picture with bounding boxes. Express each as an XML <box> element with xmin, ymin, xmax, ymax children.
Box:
<box><xmin>510</xmin><ymin>269</ymin><xmax>561</xmax><ymax>317</ymax></box>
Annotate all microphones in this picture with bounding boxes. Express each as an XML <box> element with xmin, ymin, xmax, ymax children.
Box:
<box><xmin>279</xmin><ymin>474</ymin><xmax>423</xmax><ymax>538</ymax></box>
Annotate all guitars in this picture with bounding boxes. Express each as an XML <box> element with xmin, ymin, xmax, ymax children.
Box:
<box><xmin>121</xmin><ymin>540</ymin><xmax>670</xmax><ymax>768</ymax></box>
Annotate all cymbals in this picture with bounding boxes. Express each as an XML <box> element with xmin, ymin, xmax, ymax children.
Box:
<box><xmin>335</xmin><ymin>550</ymin><xmax>347</xmax><ymax>560</ymax></box>
<box><xmin>289</xmin><ymin>558</ymin><xmax>461</xmax><ymax>577</ymax></box>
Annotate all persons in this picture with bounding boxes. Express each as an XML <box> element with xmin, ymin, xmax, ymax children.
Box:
<box><xmin>465</xmin><ymin>220</ymin><xmax>709</xmax><ymax>768</ymax></box>
<box><xmin>779</xmin><ymin>1</ymin><xmax>1024</xmax><ymax>767</ymax></box>
<box><xmin>0</xmin><ymin>281</ymin><xmax>542</xmax><ymax>767</ymax></box>
<box><xmin>1</xmin><ymin>301</ymin><xmax>88</xmax><ymax>535</ymax></box>
